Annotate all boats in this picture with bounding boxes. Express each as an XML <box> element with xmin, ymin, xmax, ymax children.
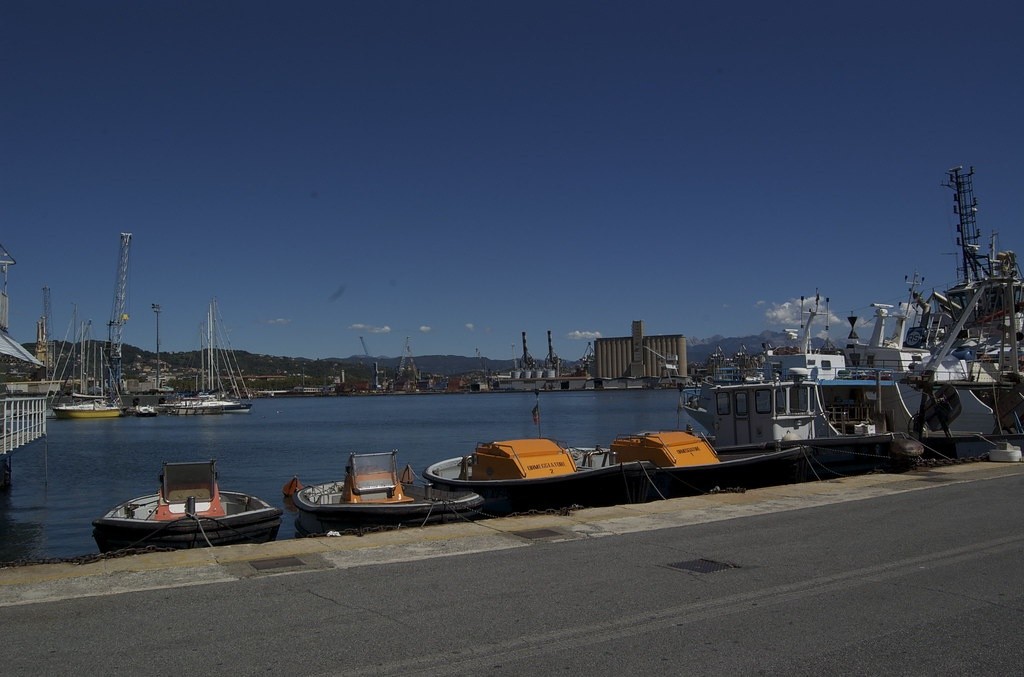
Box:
<box><xmin>0</xmin><ymin>216</ymin><xmax>782</xmax><ymax>422</ymax></box>
<box><xmin>294</xmin><ymin>449</ymin><xmax>486</xmax><ymax>539</ymax></box>
<box><xmin>681</xmin><ymin>162</ymin><xmax>1024</xmax><ymax>476</ymax></box>
<box><xmin>92</xmin><ymin>460</ymin><xmax>284</xmax><ymax>550</ymax></box>
<box><xmin>422</xmin><ymin>388</ymin><xmax>812</xmax><ymax>512</ymax></box>
